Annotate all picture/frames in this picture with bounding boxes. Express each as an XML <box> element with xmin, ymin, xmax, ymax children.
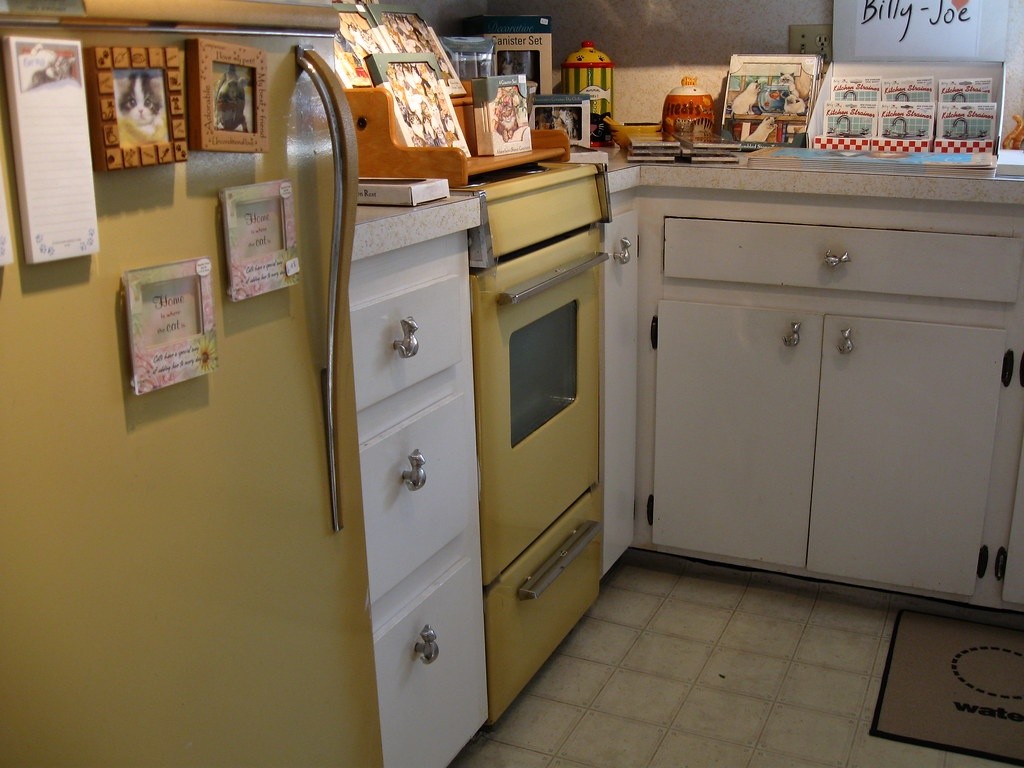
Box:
<box><xmin>83</xmin><ymin>45</ymin><xmax>190</xmax><ymax>174</ymax></box>
<box><xmin>184</xmin><ymin>39</ymin><xmax>270</xmax><ymax>153</ymax></box>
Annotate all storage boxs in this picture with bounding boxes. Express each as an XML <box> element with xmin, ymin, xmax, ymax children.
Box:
<box><xmin>332</xmin><ymin>3</ymin><xmax>468</xmax><ymax>97</ymax></box>
<box><xmin>471</xmin><ymin>74</ymin><xmax>533</xmax><ymax>157</ymax></box>
<box><xmin>358</xmin><ymin>176</ymin><xmax>451</xmax><ymax>206</ymax></box>
<box><xmin>528</xmin><ymin>94</ymin><xmax>591</xmax><ymax>151</ymax></box>
<box><xmin>363</xmin><ymin>51</ymin><xmax>471</xmax><ymax>158</ymax></box>
<box><xmin>456</xmin><ymin>13</ymin><xmax>553</xmax><ymax>97</ymax></box>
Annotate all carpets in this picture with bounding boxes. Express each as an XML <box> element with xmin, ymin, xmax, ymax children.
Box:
<box><xmin>869</xmin><ymin>609</ymin><xmax>1024</xmax><ymax>768</ymax></box>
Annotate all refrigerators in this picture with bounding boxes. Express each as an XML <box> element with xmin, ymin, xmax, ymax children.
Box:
<box><xmin>0</xmin><ymin>0</ymin><xmax>389</xmax><ymax>768</ymax></box>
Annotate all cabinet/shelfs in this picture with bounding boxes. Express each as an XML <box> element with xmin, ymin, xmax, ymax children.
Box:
<box><xmin>625</xmin><ymin>195</ymin><xmax>1024</xmax><ymax>622</ymax></box>
<box><xmin>355</xmin><ymin>233</ymin><xmax>489</xmax><ymax>768</ymax></box>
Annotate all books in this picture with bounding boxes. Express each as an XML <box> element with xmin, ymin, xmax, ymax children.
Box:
<box><xmin>626</xmin><ymin>132</ymin><xmax>741</xmax><ymax>164</ymax></box>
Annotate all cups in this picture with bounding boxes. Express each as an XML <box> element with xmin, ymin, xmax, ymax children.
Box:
<box><xmin>562</xmin><ymin>41</ymin><xmax>614</xmax><ymax>121</ymax></box>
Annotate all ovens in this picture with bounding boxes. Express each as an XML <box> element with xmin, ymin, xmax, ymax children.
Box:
<box><xmin>450</xmin><ymin>164</ymin><xmax>613</xmax><ymax>726</ymax></box>
